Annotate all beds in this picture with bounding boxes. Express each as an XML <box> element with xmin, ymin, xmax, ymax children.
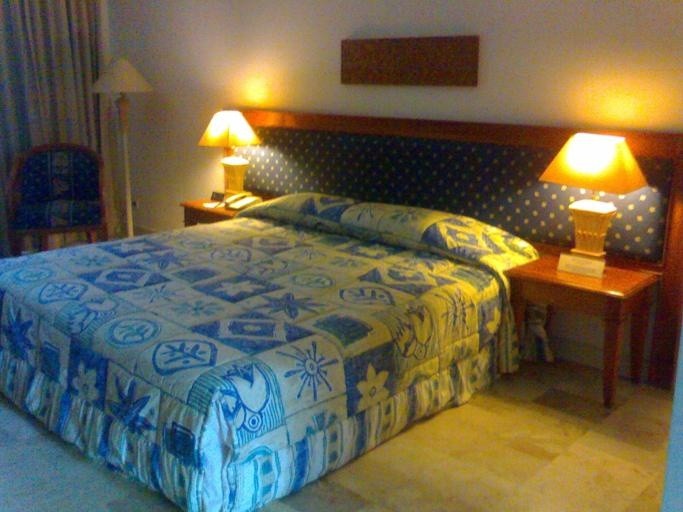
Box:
<box><xmin>0</xmin><ymin>213</ymin><xmax>550</xmax><ymax>512</ymax></box>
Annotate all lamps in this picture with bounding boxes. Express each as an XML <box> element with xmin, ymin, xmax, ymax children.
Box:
<box><xmin>538</xmin><ymin>132</ymin><xmax>648</xmax><ymax>260</ymax></box>
<box><xmin>89</xmin><ymin>57</ymin><xmax>154</xmax><ymax>237</ymax></box>
<box><xmin>197</xmin><ymin>111</ymin><xmax>262</xmax><ymax>194</ymax></box>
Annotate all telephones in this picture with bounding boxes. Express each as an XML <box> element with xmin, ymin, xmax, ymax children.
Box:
<box><xmin>225</xmin><ymin>191</ymin><xmax>262</xmax><ymax>210</ymax></box>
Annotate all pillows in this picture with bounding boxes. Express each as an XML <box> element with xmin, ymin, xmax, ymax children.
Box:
<box><xmin>238</xmin><ymin>192</ymin><xmax>537</xmax><ymax>271</ymax></box>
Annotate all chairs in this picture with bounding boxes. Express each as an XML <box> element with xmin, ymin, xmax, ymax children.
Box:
<box><xmin>3</xmin><ymin>142</ymin><xmax>112</xmax><ymax>256</ymax></box>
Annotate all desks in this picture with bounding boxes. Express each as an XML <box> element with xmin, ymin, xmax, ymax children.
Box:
<box><xmin>180</xmin><ymin>194</ymin><xmax>273</xmax><ymax>227</ymax></box>
<box><xmin>503</xmin><ymin>255</ymin><xmax>658</xmax><ymax>409</ymax></box>
